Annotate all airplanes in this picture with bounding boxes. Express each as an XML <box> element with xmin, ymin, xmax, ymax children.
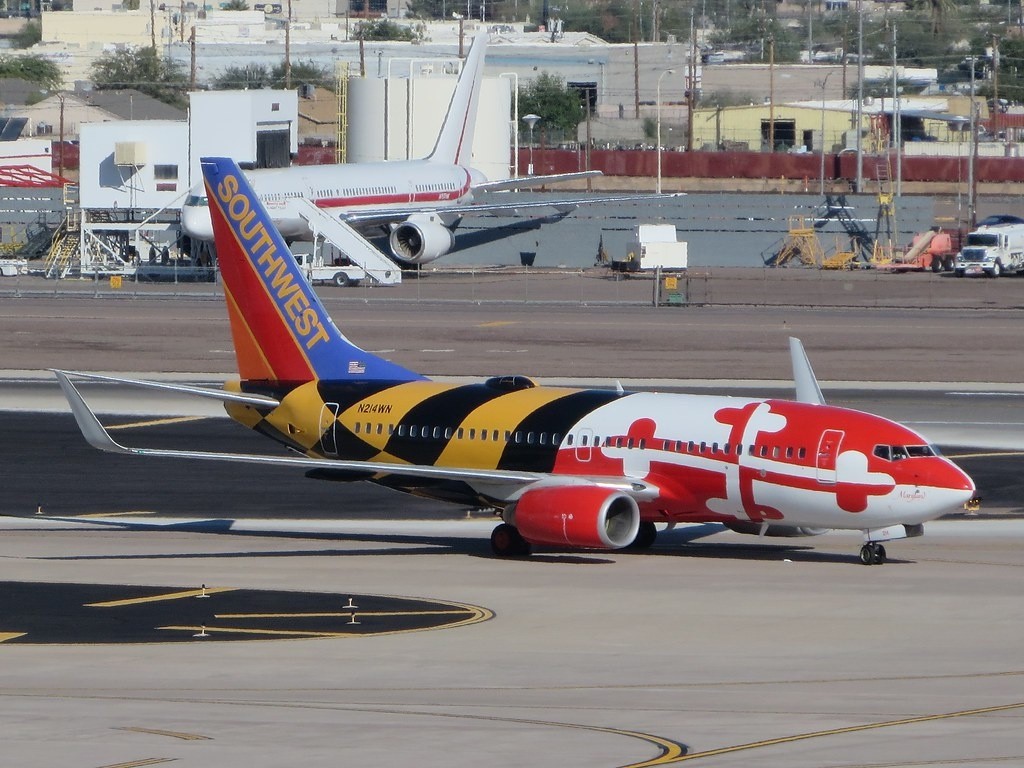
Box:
<box><xmin>175</xmin><ymin>19</ymin><xmax>606</xmax><ymax>288</ymax></box>
<box><xmin>33</xmin><ymin>152</ymin><xmax>983</xmax><ymax>564</ymax></box>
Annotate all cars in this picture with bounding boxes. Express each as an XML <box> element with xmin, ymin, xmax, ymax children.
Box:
<box><xmin>972</xmin><ymin>214</ymin><xmax>1024</xmax><ymax>228</ymax></box>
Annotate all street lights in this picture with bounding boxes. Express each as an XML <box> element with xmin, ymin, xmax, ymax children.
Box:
<box><xmin>951</xmin><ymin>90</ymin><xmax>980</xmax><ymax>229</ymax></box>
<box><xmin>779</xmin><ymin>64</ymin><xmax>843</xmax><ymax>196</ymax></box>
<box><xmin>520</xmin><ymin>113</ymin><xmax>541</xmax><ymax>194</ymax></box>
<box><xmin>655</xmin><ymin>69</ymin><xmax>677</xmax><ymax>196</ymax></box>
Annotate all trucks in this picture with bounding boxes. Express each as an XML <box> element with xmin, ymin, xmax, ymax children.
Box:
<box><xmin>951</xmin><ymin>223</ymin><xmax>1024</xmax><ymax>278</ymax></box>
